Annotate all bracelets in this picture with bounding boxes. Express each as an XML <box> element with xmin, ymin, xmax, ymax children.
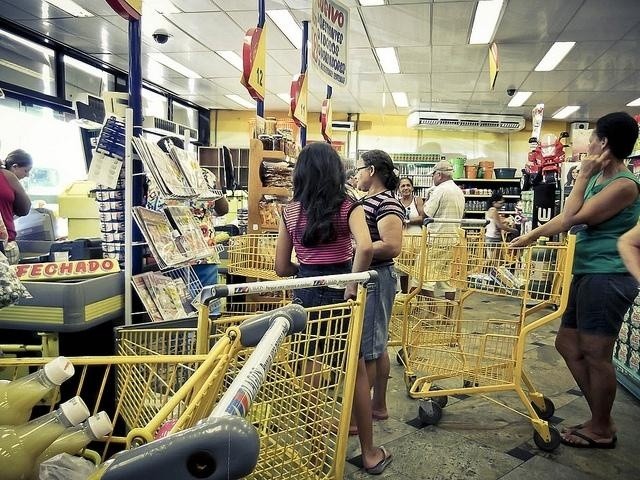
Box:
<box><xmin>405</xmin><ymin>219</ymin><xmax>410</xmax><ymax>224</ymax></box>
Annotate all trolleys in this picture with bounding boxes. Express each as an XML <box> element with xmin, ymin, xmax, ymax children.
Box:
<box><xmin>0</xmin><ymin>300</ymin><xmax>316</xmax><ymax>480</ymax></box>
<box><xmin>114</xmin><ymin>268</ymin><xmax>383</xmax><ymax>479</ymax></box>
<box><xmin>399</xmin><ymin>230</ymin><xmax>579</xmax><ymax>455</ymax></box>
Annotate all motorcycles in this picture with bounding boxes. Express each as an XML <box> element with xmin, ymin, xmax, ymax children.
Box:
<box><xmin>519</xmin><ymin>131</ymin><xmax>570</xmax><ymax>189</ymax></box>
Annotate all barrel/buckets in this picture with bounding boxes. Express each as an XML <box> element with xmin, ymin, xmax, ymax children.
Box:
<box><xmin>463</xmin><ymin>165</ymin><xmax>478</xmax><ymax>179</ymax></box>
<box><xmin>478</xmin><ymin>160</ymin><xmax>495</xmax><ymax>179</ymax></box>
<box><xmin>449</xmin><ymin>157</ymin><xmax>465</xmax><ymax>178</ymax></box>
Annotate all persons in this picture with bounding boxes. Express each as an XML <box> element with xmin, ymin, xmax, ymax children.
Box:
<box><xmin>344</xmin><ymin>169</ymin><xmax>361</xmax><ymax>202</ymax></box>
<box><xmin>323</xmin><ymin>150</ymin><xmax>407</xmax><ymax>435</ymax></box>
<box><xmin>398</xmin><ymin>177</ymin><xmax>427</xmax><ymax>295</ymax></box>
<box><xmin>510</xmin><ymin>112</ymin><xmax>640</xmax><ymax>450</ymax></box>
<box><xmin>415</xmin><ymin>160</ymin><xmax>465</xmax><ymax>326</ymax></box>
<box><xmin>484</xmin><ymin>193</ymin><xmax>519</xmax><ymax>267</ymax></box>
<box><xmin>616</xmin><ymin>224</ymin><xmax>640</xmax><ymax>283</ymax></box>
<box><xmin>564</xmin><ymin>165</ymin><xmax>579</xmax><ymax>188</ymax></box>
<box><xmin>0</xmin><ymin>149</ymin><xmax>32</xmax><ymax>265</ymax></box>
<box><xmin>274</xmin><ymin>142</ymin><xmax>393</xmax><ymax>475</ymax></box>
<box><xmin>145</xmin><ymin>137</ymin><xmax>229</xmax><ymax>317</ymax></box>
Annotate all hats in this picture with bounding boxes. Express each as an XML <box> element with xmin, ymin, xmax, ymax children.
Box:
<box><xmin>425</xmin><ymin>160</ymin><xmax>456</xmax><ymax>177</ymax></box>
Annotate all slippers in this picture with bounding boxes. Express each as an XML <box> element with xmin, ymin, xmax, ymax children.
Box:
<box><xmin>372</xmin><ymin>407</ymin><xmax>392</xmax><ymax>419</ymax></box>
<box><xmin>364</xmin><ymin>446</ymin><xmax>395</xmax><ymax>475</ymax></box>
<box><xmin>321</xmin><ymin>420</ymin><xmax>361</xmax><ymax>435</ymax></box>
<box><xmin>558</xmin><ymin>422</ymin><xmax>618</xmax><ymax>450</ymax></box>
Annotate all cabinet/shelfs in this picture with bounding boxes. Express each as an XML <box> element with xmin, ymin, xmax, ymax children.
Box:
<box><xmin>390</xmin><ymin>161</ymin><xmax>437</xmax><ymax>202</ymax></box>
<box><xmin>120</xmin><ymin>110</ymin><xmax>226</xmax><ymax>325</ymax></box>
<box><xmin>246</xmin><ymin>138</ymin><xmax>295</xmax><ymax>311</ymax></box>
<box><xmin>452</xmin><ymin>178</ymin><xmax>522</xmax><ymax>235</ymax></box>
<box><xmin>196</xmin><ymin>145</ymin><xmax>248</xmax><ymax>190</ymax></box>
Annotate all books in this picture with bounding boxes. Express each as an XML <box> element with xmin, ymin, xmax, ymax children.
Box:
<box><xmin>129</xmin><ymin>134</ymin><xmax>215</xmax><ymax>323</ymax></box>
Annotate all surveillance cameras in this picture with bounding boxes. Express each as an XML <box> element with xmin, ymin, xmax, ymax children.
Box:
<box><xmin>152</xmin><ymin>30</ymin><xmax>170</xmax><ymax>44</ymax></box>
<box><xmin>507</xmin><ymin>87</ymin><xmax>517</xmax><ymax>96</ymax></box>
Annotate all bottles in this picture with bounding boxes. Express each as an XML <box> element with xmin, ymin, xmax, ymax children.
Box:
<box><xmin>465</xmin><ymin>200</ymin><xmax>487</xmax><ymax>211</ymax></box>
<box><xmin>0</xmin><ymin>355</ymin><xmax>113</xmax><ymax>479</ymax></box>
<box><xmin>258</xmin><ymin>133</ymin><xmax>297</xmax><ymax>159</ymax></box>
<box><xmin>387</xmin><ymin>152</ymin><xmax>441</xmax><ymax>200</ymax></box>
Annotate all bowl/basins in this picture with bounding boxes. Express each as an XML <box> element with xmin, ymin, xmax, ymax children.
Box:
<box><xmin>494</xmin><ymin>168</ymin><xmax>516</xmax><ymax>178</ymax></box>
